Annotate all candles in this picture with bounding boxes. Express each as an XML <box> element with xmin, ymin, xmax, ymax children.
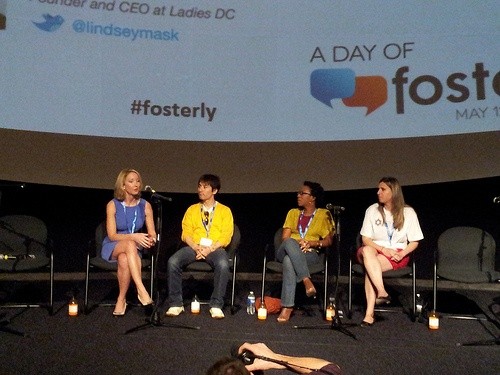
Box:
<box><xmin>258</xmin><ymin>305</ymin><xmax>267</xmax><ymax>320</ymax></box>
<box><xmin>191</xmin><ymin>299</ymin><xmax>200</xmax><ymax>313</ymax></box>
<box><xmin>326</xmin><ymin>307</ymin><xmax>335</xmax><ymax>320</ymax></box>
<box><xmin>429</xmin><ymin>315</ymin><xmax>439</xmax><ymax>329</ymax></box>
<box><xmin>69</xmin><ymin>302</ymin><xmax>78</xmax><ymax>315</ymax></box>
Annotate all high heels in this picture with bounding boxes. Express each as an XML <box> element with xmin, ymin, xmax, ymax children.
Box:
<box><xmin>277</xmin><ymin>307</ymin><xmax>294</xmax><ymax>322</ymax></box>
<box><xmin>137</xmin><ymin>295</ymin><xmax>156</xmax><ymax>310</ymax></box>
<box><xmin>306</xmin><ymin>286</ymin><xmax>316</xmax><ymax>299</ymax></box>
<box><xmin>376</xmin><ymin>295</ymin><xmax>392</xmax><ymax>305</ymax></box>
<box><xmin>360</xmin><ymin>320</ymin><xmax>375</xmax><ymax>327</ymax></box>
<box><xmin>112</xmin><ymin>302</ymin><xmax>127</xmax><ymax>319</ymax></box>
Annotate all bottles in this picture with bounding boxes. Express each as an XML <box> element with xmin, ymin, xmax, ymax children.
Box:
<box><xmin>246</xmin><ymin>291</ymin><xmax>256</xmax><ymax>316</ymax></box>
<box><xmin>428</xmin><ymin>311</ymin><xmax>440</xmax><ymax>330</ymax></box>
<box><xmin>326</xmin><ymin>296</ymin><xmax>336</xmax><ymax>321</ymax></box>
<box><xmin>68</xmin><ymin>298</ymin><xmax>78</xmax><ymax>316</ymax></box>
<box><xmin>190</xmin><ymin>298</ymin><xmax>201</xmax><ymax>314</ymax></box>
<box><xmin>415</xmin><ymin>294</ymin><xmax>424</xmax><ymax>319</ymax></box>
<box><xmin>257</xmin><ymin>300</ymin><xmax>268</xmax><ymax>321</ymax></box>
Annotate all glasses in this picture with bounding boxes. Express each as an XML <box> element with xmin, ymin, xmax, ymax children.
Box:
<box><xmin>204</xmin><ymin>211</ymin><xmax>209</xmax><ymax>225</ymax></box>
<box><xmin>297</xmin><ymin>190</ymin><xmax>314</xmax><ymax>197</ymax></box>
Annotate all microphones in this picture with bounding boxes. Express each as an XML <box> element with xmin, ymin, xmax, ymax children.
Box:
<box><xmin>325</xmin><ymin>203</ymin><xmax>345</xmax><ymax>211</ymax></box>
<box><xmin>145</xmin><ymin>185</ymin><xmax>156</xmax><ymax>193</ymax></box>
<box><xmin>0</xmin><ymin>254</ymin><xmax>17</xmax><ymax>260</ymax></box>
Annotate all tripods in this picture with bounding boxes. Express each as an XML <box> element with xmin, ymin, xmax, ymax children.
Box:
<box><xmin>121</xmin><ymin>195</ymin><xmax>201</xmax><ymax>335</ymax></box>
<box><xmin>292</xmin><ymin>210</ymin><xmax>359</xmax><ymax>340</ymax></box>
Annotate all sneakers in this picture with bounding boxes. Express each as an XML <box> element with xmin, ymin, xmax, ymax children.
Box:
<box><xmin>165</xmin><ymin>306</ymin><xmax>184</xmax><ymax>317</ymax></box>
<box><xmin>209</xmin><ymin>306</ymin><xmax>225</xmax><ymax>319</ymax></box>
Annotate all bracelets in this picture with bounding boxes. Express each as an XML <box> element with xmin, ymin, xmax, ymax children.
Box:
<box><xmin>316</xmin><ymin>240</ymin><xmax>320</xmax><ymax>248</ymax></box>
<box><xmin>380</xmin><ymin>246</ymin><xmax>385</xmax><ymax>253</ymax></box>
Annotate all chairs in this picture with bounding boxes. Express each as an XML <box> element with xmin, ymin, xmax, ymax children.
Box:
<box><xmin>348</xmin><ymin>230</ymin><xmax>416</xmax><ymax>316</ymax></box>
<box><xmin>85</xmin><ymin>222</ymin><xmax>154</xmax><ymax>306</ymax></box>
<box><xmin>0</xmin><ymin>216</ymin><xmax>53</xmax><ymax>314</ymax></box>
<box><xmin>185</xmin><ymin>223</ymin><xmax>241</xmax><ymax>315</ymax></box>
<box><xmin>434</xmin><ymin>226</ymin><xmax>500</xmax><ymax>320</ymax></box>
<box><xmin>263</xmin><ymin>228</ymin><xmax>328</xmax><ymax>312</ymax></box>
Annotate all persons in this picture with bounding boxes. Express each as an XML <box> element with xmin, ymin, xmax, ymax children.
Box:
<box><xmin>208</xmin><ymin>342</ymin><xmax>343</xmax><ymax>375</ymax></box>
<box><xmin>357</xmin><ymin>176</ymin><xmax>424</xmax><ymax>325</ymax></box>
<box><xmin>165</xmin><ymin>174</ymin><xmax>234</xmax><ymax>319</ymax></box>
<box><xmin>100</xmin><ymin>168</ymin><xmax>156</xmax><ymax>315</ymax></box>
<box><xmin>276</xmin><ymin>181</ymin><xmax>335</xmax><ymax>322</ymax></box>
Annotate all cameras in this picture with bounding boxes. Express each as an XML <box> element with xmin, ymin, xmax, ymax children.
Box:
<box><xmin>231</xmin><ymin>340</ymin><xmax>256</xmax><ymax>365</ymax></box>
